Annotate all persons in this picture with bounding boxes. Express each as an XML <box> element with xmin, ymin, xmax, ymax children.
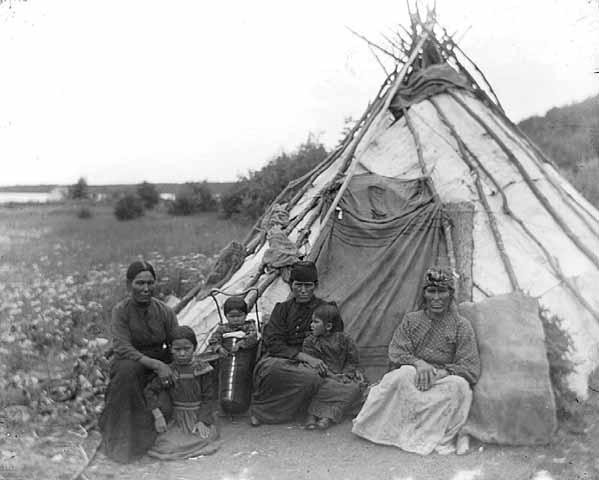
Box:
<box><xmin>298</xmin><ymin>306</ymin><xmax>364</xmax><ymax>429</ymax></box>
<box><xmin>99</xmin><ymin>261</ymin><xmax>180</xmax><ymax>464</ymax></box>
<box><xmin>207</xmin><ymin>296</ymin><xmax>258</xmax><ymax>416</ymax></box>
<box><xmin>250</xmin><ymin>262</ymin><xmax>337</xmax><ymax>424</ymax></box>
<box><xmin>144</xmin><ymin>326</ymin><xmax>223</xmax><ymax>460</ymax></box>
<box><xmin>352</xmin><ymin>266</ymin><xmax>483</xmax><ymax>457</ymax></box>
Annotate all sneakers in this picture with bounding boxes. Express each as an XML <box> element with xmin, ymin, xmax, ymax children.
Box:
<box><xmin>317</xmin><ymin>418</ymin><xmax>330</xmax><ymax>430</ymax></box>
<box><xmin>305</xmin><ymin>416</ymin><xmax>317</xmax><ymax>431</ymax></box>
<box><xmin>250</xmin><ymin>414</ymin><xmax>261</xmax><ymax>426</ymax></box>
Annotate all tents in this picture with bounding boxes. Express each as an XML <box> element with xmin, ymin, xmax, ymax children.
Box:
<box><xmin>161</xmin><ymin>59</ymin><xmax>598</xmax><ymax>445</ymax></box>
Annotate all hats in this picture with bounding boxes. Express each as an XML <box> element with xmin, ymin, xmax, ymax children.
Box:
<box><xmin>423</xmin><ymin>265</ymin><xmax>457</xmax><ymax>290</ymax></box>
<box><xmin>289</xmin><ymin>260</ymin><xmax>319</xmax><ymax>282</ymax></box>
